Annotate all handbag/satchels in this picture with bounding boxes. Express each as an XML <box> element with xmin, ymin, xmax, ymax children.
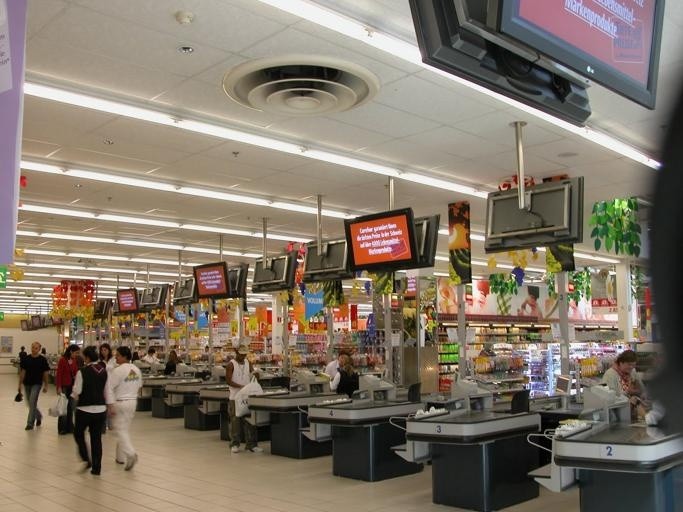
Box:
<box><xmin>634</xmin><ymin>397</ymin><xmax>665</xmax><ymax>425</ymax></box>
<box><xmin>234</xmin><ymin>376</ymin><xmax>263</xmax><ymax>417</ymax></box>
<box><xmin>47</xmin><ymin>393</ymin><xmax>69</xmax><ymax>417</ymax></box>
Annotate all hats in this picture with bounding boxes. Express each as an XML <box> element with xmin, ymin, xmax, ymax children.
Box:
<box><xmin>236</xmin><ymin>344</ymin><xmax>248</xmax><ymax>355</ymax></box>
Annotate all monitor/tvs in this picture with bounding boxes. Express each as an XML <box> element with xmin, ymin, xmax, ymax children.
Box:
<box><xmin>556</xmin><ymin>374</ymin><xmax>572</xmax><ymax>397</ymax></box>
<box><xmin>302</xmin><ymin>237</ymin><xmax>356</xmax><ymax>284</ymax></box>
<box><xmin>484</xmin><ymin>176</ymin><xmax>584</xmax><ymax>253</ymax></box>
<box><xmin>485</xmin><ymin>0</ymin><xmax>665</xmax><ymax>110</ymax></box>
<box><xmin>20</xmin><ymin>278</ymin><xmax>198</xmax><ymax>332</ymax></box>
<box><xmin>228</xmin><ymin>263</ymin><xmax>250</xmax><ymax>298</ymax></box>
<box><xmin>408</xmin><ymin>0</ymin><xmax>592</xmax><ymax>128</ymax></box>
<box><xmin>252</xmin><ymin>250</ymin><xmax>298</xmax><ymax>293</ymax></box>
<box><xmin>193</xmin><ymin>262</ymin><xmax>230</xmax><ymax>298</ymax></box>
<box><xmin>344</xmin><ymin>207</ymin><xmax>440</xmax><ymax>270</ymax></box>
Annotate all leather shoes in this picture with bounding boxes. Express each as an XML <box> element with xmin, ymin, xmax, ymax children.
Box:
<box><xmin>25</xmin><ymin>426</ymin><xmax>32</xmax><ymax>430</ymax></box>
<box><xmin>36</xmin><ymin>415</ymin><xmax>40</xmax><ymax>426</ymax></box>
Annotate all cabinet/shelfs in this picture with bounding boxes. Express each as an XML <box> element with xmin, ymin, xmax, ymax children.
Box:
<box><xmin>432</xmin><ymin>323</ymin><xmax>632</xmax><ymax>396</ymax></box>
<box><xmin>248</xmin><ymin>330</ymin><xmax>391</xmax><ymax>381</ymax></box>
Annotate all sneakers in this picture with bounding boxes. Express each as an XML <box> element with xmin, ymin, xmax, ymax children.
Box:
<box><xmin>231</xmin><ymin>446</ymin><xmax>238</xmax><ymax>453</ymax></box>
<box><xmin>248</xmin><ymin>447</ymin><xmax>263</xmax><ymax>452</ymax></box>
<box><xmin>124</xmin><ymin>455</ymin><xmax>136</xmax><ymax>471</ymax></box>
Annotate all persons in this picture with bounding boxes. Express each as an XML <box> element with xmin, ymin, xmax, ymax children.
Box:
<box><xmin>325</xmin><ymin>352</ymin><xmax>350</xmax><ymax>379</ymax></box>
<box><xmin>14</xmin><ymin>341</ymin><xmax>212</xmax><ymax>475</ymax></box>
<box><xmin>329</xmin><ymin>357</ymin><xmax>359</xmax><ymax>398</ymax></box>
<box><xmin>600</xmin><ymin>350</ymin><xmax>647</xmax><ymax>424</ymax></box>
<box><xmin>226</xmin><ymin>345</ymin><xmax>264</xmax><ymax>453</ymax></box>
